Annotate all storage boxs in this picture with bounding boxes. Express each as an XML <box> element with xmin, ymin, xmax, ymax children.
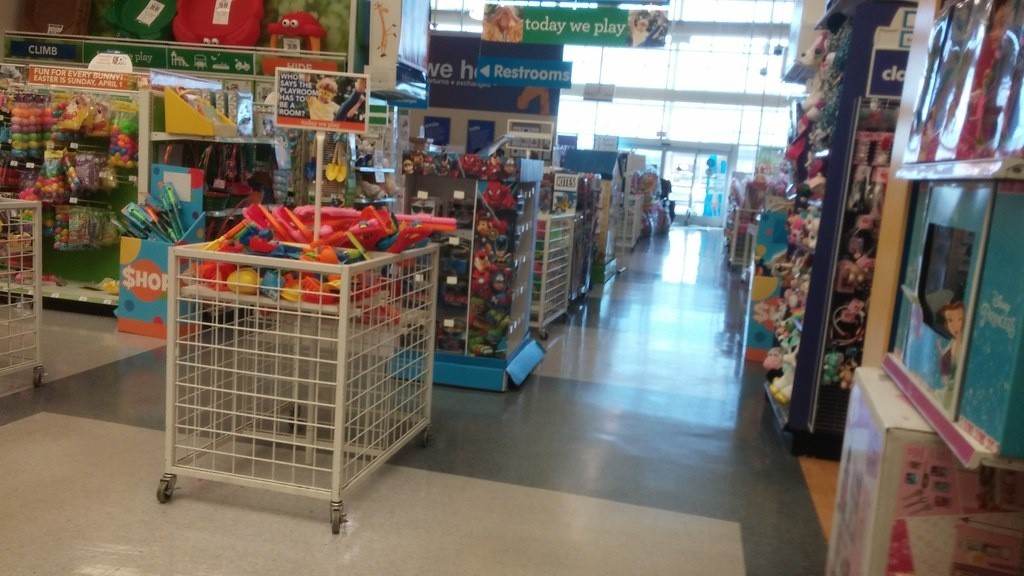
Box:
<box><xmin>822</xmin><ymin>366</ymin><xmax>1024</xmax><ymax>576</ymax></box>
<box><xmin>228</xmin><ymin>91</ymin><xmax>253</xmax><ymax>136</ymax></box>
<box><xmin>202</xmin><ymin>89</ymin><xmax>215</xmax><ymax>106</ymax></box>
<box><xmin>253</xmin><ymin>112</ymin><xmax>274</xmax><ymax>136</ymax></box>
<box><xmin>894</xmin><ymin>182</ymin><xmax>1024</xmax><ymax>461</ymax></box>
<box><xmin>193</xmin><ymin>88</ymin><xmax>201</xmax><ymax>96</ymax></box>
<box><xmin>253</xmin><ymin>104</ymin><xmax>274</xmax><ymax>112</ymax></box>
<box><xmin>215</xmin><ymin>89</ymin><xmax>228</xmax><ymax>117</ymax></box>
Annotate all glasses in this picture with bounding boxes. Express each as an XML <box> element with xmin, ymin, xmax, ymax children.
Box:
<box><xmin>413</xmin><ymin>206</ymin><xmax>433</xmax><ymax>213</ymax></box>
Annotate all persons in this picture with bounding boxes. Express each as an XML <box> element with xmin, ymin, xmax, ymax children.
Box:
<box><xmin>307</xmin><ymin>79</ymin><xmax>364</xmax><ymax>121</ymax></box>
<box><xmin>493</xmin><ymin>5</ymin><xmax>521</xmax><ymax>41</ymax></box>
<box><xmin>629</xmin><ymin>10</ymin><xmax>666</xmax><ymax>47</ymax></box>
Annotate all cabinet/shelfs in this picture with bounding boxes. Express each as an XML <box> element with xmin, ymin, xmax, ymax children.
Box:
<box><xmin>528</xmin><ymin>212</ymin><xmax>575</xmax><ymax>341</ymax></box>
